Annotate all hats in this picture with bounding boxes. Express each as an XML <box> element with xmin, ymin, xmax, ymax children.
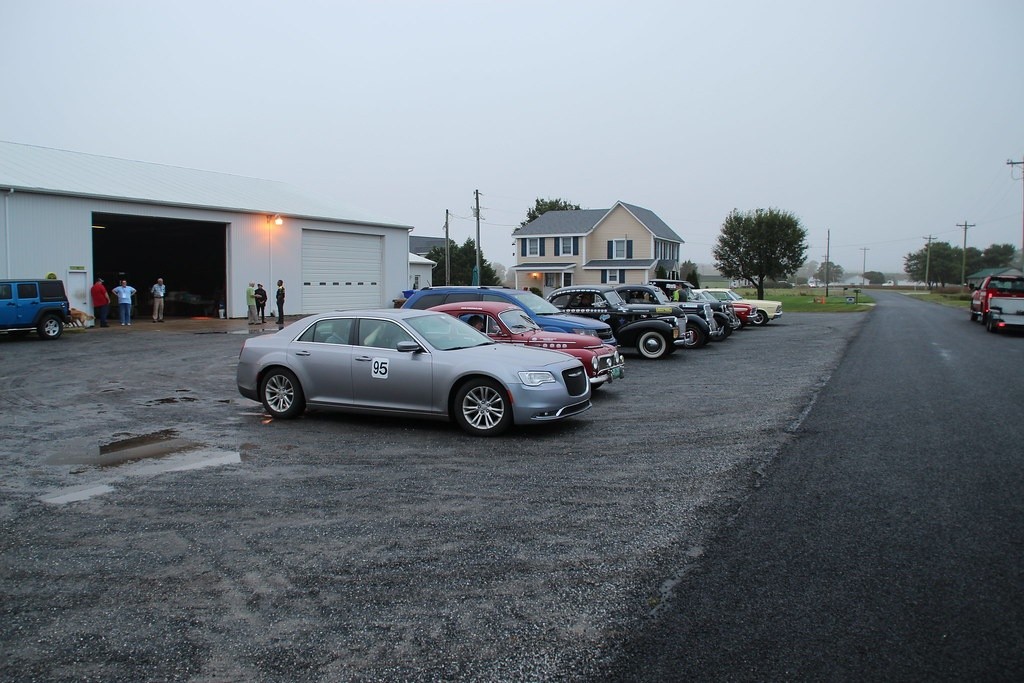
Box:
<box><xmin>257</xmin><ymin>282</ymin><xmax>263</xmax><ymax>287</ymax></box>
<box><xmin>97</xmin><ymin>277</ymin><xmax>105</xmax><ymax>283</ymax></box>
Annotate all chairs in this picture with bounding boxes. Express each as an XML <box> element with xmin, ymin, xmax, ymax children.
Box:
<box><xmin>568</xmin><ymin>297</ymin><xmax>579</xmax><ymax>307</ymax></box>
<box><xmin>308</xmin><ymin>330</ymin><xmax>325</xmax><ymax>342</ymax></box>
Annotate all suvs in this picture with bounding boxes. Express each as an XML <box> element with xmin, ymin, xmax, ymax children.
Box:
<box><xmin>398</xmin><ymin>285</ymin><xmax>620</xmax><ymax>350</ymax></box>
<box><xmin>0</xmin><ymin>278</ymin><xmax>72</xmax><ymax>340</ymax></box>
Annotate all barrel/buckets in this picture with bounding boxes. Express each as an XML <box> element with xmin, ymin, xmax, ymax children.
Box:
<box><xmin>219</xmin><ymin>309</ymin><xmax>226</xmax><ymax>318</ymax></box>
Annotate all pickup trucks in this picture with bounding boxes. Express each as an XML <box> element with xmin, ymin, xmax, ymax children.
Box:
<box><xmin>968</xmin><ymin>275</ymin><xmax>1024</xmax><ymax>326</ymax></box>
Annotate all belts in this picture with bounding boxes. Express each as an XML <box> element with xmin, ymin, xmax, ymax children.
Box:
<box><xmin>154</xmin><ymin>297</ymin><xmax>163</xmax><ymax>298</ymax></box>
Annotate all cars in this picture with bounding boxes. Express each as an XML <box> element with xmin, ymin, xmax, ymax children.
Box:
<box><xmin>614</xmin><ymin>285</ymin><xmax>725</xmax><ymax>349</ymax></box>
<box><xmin>544</xmin><ymin>286</ymin><xmax>695</xmax><ymax>361</ymax></box>
<box><xmin>691</xmin><ymin>289</ymin><xmax>758</xmax><ymax>330</ymax></box>
<box><xmin>236</xmin><ymin>308</ymin><xmax>593</xmax><ymax>438</ymax></box>
<box><xmin>648</xmin><ymin>279</ymin><xmax>741</xmax><ymax>342</ymax></box>
<box><xmin>364</xmin><ymin>301</ymin><xmax>626</xmax><ymax>391</ymax></box>
<box><xmin>706</xmin><ymin>289</ymin><xmax>783</xmax><ymax>326</ymax></box>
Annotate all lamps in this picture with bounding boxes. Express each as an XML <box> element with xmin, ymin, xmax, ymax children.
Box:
<box><xmin>266</xmin><ymin>214</ymin><xmax>282</xmax><ymax>225</ymax></box>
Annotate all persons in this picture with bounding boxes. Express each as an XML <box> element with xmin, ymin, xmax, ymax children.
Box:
<box><xmin>467</xmin><ymin>315</ymin><xmax>483</xmax><ymax>330</ymax></box>
<box><xmin>246</xmin><ymin>282</ymin><xmax>262</xmax><ymax>325</ymax></box>
<box><xmin>326</xmin><ymin>322</ymin><xmax>388</xmax><ymax>346</ymax></box>
<box><xmin>112</xmin><ymin>280</ymin><xmax>137</xmax><ymax>325</ymax></box>
<box><xmin>255</xmin><ymin>283</ymin><xmax>267</xmax><ymax>323</ymax></box>
<box><xmin>673</xmin><ymin>284</ymin><xmax>687</xmax><ymax>302</ymax></box>
<box><xmin>151</xmin><ymin>279</ymin><xmax>166</xmax><ymax>323</ymax></box>
<box><xmin>90</xmin><ymin>278</ymin><xmax>111</xmax><ymax>327</ymax></box>
<box><xmin>276</xmin><ymin>280</ymin><xmax>285</xmax><ymax>324</ymax></box>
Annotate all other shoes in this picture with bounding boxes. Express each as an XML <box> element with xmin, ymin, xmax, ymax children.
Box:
<box><xmin>254</xmin><ymin>323</ymin><xmax>261</xmax><ymax>325</ymax></box>
<box><xmin>157</xmin><ymin>320</ymin><xmax>164</xmax><ymax>322</ymax></box>
<box><xmin>126</xmin><ymin>322</ymin><xmax>131</xmax><ymax>326</ymax></box>
<box><xmin>262</xmin><ymin>322</ymin><xmax>266</xmax><ymax>323</ymax></box>
<box><xmin>248</xmin><ymin>323</ymin><xmax>254</xmax><ymax>325</ymax></box>
<box><xmin>121</xmin><ymin>322</ymin><xmax>126</xmax><ymax>326</ymax></box>
<box><xmin>100</xmin><ymin>324</ymin><xmax>110</xmax><ymax>328</ymax></box>
<box><xmin>152</xmin><ymin>321</ymin><xmax>156</xmax><ymax>323</ymax></box>
<box><xmin>276</xmin><ymin>321</ymin><xmax>283</xmax><ymax>324</ymax></box>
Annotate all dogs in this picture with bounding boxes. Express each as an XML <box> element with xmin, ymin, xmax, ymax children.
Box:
<box><xmin>70</xmin><ymin>308</ymin><xmax>96</xmax><ymax>328</ymax></box>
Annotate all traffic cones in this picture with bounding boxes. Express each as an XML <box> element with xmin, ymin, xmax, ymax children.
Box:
<box><xmin>822</xmin><ymin>296</ymin><xmax>825</xmax><ymax>304</ymax></box>
